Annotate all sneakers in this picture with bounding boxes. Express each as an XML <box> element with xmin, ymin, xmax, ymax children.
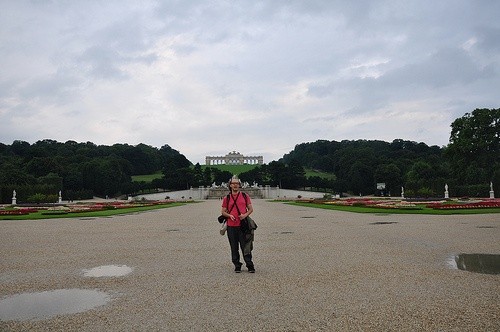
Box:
<box><xmin>234</xmin><ymin>266</ymin><xmax>241</xmax><ymax>273</ymax></box>
<box><xmin>248</xmin><ymin>267</ymin><xmax>255</xmax><ymax>273</ymax></box>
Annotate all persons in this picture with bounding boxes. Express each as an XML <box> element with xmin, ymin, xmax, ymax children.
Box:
<box><xmin>217</xmin><ymin>179</ymin><xmax>258</xmax><ymax>273</ymax></box>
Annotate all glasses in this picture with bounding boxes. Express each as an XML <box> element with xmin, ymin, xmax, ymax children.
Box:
<box><xmin>231</xmin><ymin>183</ymin><xmax>240</xmax><ymax>185</ymax></box>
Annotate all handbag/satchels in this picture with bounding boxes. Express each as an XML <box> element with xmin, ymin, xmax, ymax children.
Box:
<box><xmin>218</xmin><ymin>215</ymin><xmax>228</xmax><ymax>224</ymax></box>
<box><xmin>241</xmin><ymin>217</ymin><xmax>257</xmax><ymax>230</ymax></box>
<box><xmin>220</xmin><ymin>220</ymin><xmax>227</xmax><ymax>235</ymax></box>
